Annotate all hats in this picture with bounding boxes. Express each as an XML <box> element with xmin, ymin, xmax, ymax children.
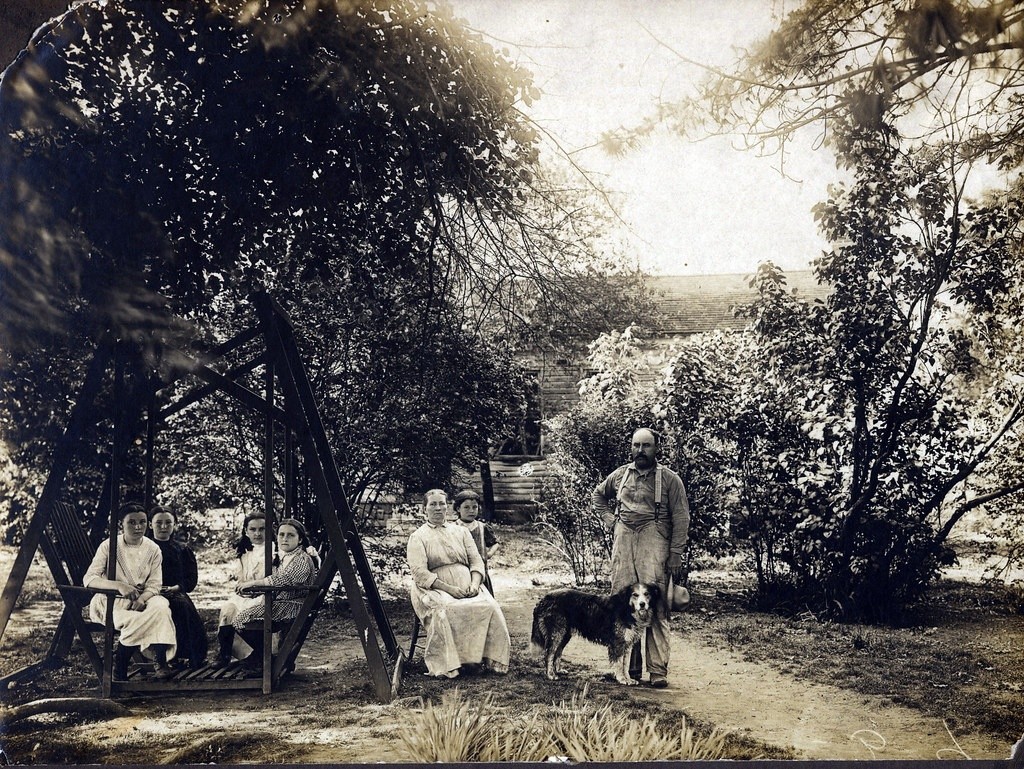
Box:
<box><xmin>668</xmin><ymin>575</ymin><xmax>690</xmax><ymax>618</ymax></box>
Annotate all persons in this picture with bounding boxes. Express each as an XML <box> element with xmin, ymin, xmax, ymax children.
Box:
<box><xmin>233</xmin><ymin>520</ymin><xmax>315</xmax><ymax>669</ymax></box>
<box><xmin>407</xmin><ymin>488</ymin><xmax>510</xmax><ymax>677</ymax></box>
<box><xmin>211</xmin><ymin>511</ymin><xmax>322</xmax><ymax>669</ymax></box>
<box><xmin>83</xmin><ymin>502</ymin><xmax>177</xmax><ymax>681</ymax></box>
<box><xmin>453</xmin><ymin>489</ymin><xmax>500</xmax><ymax>599</ymax></box>
<box><xmin>590</xmin><ymin>429</ymin><xmax>689</xmax><ymax>689</ymax></box>
<box><xmin>147</xmin><ymin>506</ymin><xmax>211</xmax><ymax>669</ymax></box>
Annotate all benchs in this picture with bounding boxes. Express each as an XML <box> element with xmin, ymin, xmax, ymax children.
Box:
<box><xmin>38</xmin><ymin>500</ymin><xmax>155</xmax><ymax>680</ymax></box>
<box><xmin>238</xmin><ymin>530</ymin><xmax>353</xmax><ymax>679</ymax></box>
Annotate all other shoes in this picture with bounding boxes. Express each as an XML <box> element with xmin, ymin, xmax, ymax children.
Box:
<box><xmin>650</xmin><ymin>673</ymin><xmax>668</xmax><ymax>688</ymax></box>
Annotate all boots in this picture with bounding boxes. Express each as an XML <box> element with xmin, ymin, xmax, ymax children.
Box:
<box><xmin>153</xmin><ymin>647</ymin><xmax>172</xmax><ymax>676</ymax></box>
<box><xmin>211</xmin><ymin>624</ymin><xmax>235</xmax><ymax>668</ymax></box>
<box><xmin>114</xmin><ymin>641</ymin><xmax>137</xmax><ymax>680</ymax></box>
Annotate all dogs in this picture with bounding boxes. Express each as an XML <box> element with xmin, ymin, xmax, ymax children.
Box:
<box><xmin>528</xmin><ymin>582</ymin><xmax>663</xmax><ymax>689</ymax></box>
